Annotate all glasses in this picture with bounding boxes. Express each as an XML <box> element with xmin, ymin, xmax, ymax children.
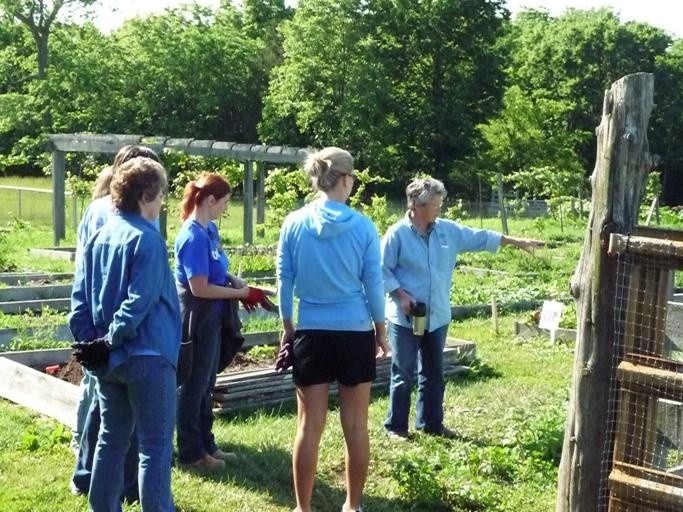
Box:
<box><xmin>349</xmin><ymin>174</ymin><xmax>356</xmax><ymax>181</ymax></box>
<box><xmin>431</xmin><ymin>203</ymin><xmax>443</xmax><ymax>209</ymax></box>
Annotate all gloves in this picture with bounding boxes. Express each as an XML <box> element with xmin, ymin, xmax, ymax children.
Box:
<box><xmin>243</xmin><ymin>287</ymin><xmax>278</xmax><ymax>312</ymax></box>
<box><xmin>275</xmin><ymin>338</ymin><xmax>293</xmax><ymax>374</ymax></box>
<box><xmin>70</xmin><ymin>338</ymin><xmax>112</xmax><ymax>371</ymax></box>
<box><xmin>241</xmin><ymin>302</ymin><xmax>257</xmax><ymax>314</ymax></box>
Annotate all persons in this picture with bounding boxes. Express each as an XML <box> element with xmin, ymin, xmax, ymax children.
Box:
<box><xmin>70</xmin><ymin>167</ymin><xmax>113</xmax><ymax>457</ymax></box>
<box><xmin>380</xmin><ymin>177</ymin><xmax>545</xmax><ymax>442</ymax></box>
<box><xmin>174</xmin><ymin>174</ymin><xmax>249</xmax><ymax>472</ymax></box>
<box><xmin>69</xmin><ymin>145</ymin><xmax>160</xmax><ymax>498</ymax></box>
<box><xmin>276</xmin><ymin>147</ymin><xmax>389</xmax><ymax>512</ymax></box>
<box><xmin>70</xmin><ymin>158</ymin><xmax>180</xmax><ymax>511</ymax></box>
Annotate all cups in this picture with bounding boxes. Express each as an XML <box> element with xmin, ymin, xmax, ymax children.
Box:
<box><xmin>405</xmin><ymin>302</ymin><xmax>427</xmax><ymax>336</ymax></box>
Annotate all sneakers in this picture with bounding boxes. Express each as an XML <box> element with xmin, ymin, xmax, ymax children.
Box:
<box><xmin>213</xmin><ymin>450</ymin><xmax>236</xmax><ymax>464</ymax></box>
<box><xmin>421</xmin><ymin>424</ymin><xmax>457</xmax><ymax>440</ymax></box>
<box><xmin>179</xmin><ymin>455</ymin><xmax>226</xmax><ymax>470</ymax></box>
<box><xmin>386</xmin><ymin>430</ymin><xmax>409</xmax><ymax>443</ymax></box>
